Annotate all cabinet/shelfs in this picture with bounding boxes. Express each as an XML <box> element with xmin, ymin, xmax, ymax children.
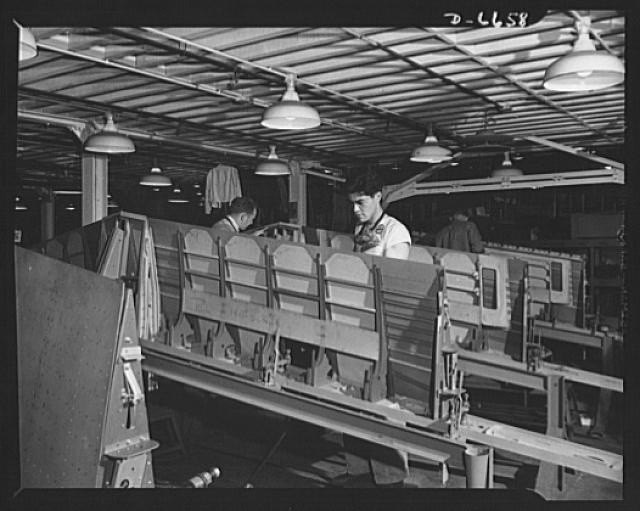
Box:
<box><xmin>511</xmin><ymin>238</ymin><xmax>626</xmax><ymax>335</ymax></box>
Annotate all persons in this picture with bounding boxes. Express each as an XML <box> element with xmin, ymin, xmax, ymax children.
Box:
<box><xmin>344</xmin><ymin>172</ymin><xmax>411</xmax><ymax>261</ymax></box>
<box><xmin>211</xmin><ymin>196</ymin><xmax>258</xmax><ymax>234</ymax></box>
<box><xmin>435</xmin><ymin>209</ymin><xmax>484</xmax><ymax>254</ymax></box>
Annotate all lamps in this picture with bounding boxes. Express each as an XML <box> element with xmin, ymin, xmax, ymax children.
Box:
<box><xmin>139</xmin><ymin>159</ymin><xmax>173</xmax><ymax>192</ymax></box>
<box><xmin>252</xmin><ymin>141</ymin><xmax>293</xmax><ymax>177</ymax></box>
<box><xmin>83</xmin><ymin>110</ymin><xmax>140</xmax><ymax>156</ymax></box>
<box><xmin>409</xmin><ymin>119</ymin><xmax>456</xmax><ymax>166</ymax></box>
<box><xmin>543</xmin><ymin>18</ymin><xmax>627</xmax><ymax>94</ymax></box>
<box><xmin>489</xmin><ymin>150</ymin><xmax>525</xmax><ymax>181</ymax></box>
<box><xmin>18</xmin><ymin>27</ymin><xmax>43</xmax><ymax>60</ymax></box>
<box><xmin>259</xmin><ymin>71</ymin><xmax>324</xmax><ymax>133</ymax></box>
